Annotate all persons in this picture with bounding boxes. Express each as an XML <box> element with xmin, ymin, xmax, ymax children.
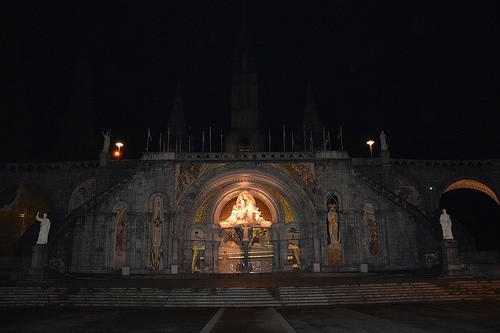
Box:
<box><xmin>151</xmin><ymin>204</ymin><xmax>164</xmax><ymax>269</ymax></box>
<box><xmin>35</xmin><ymin>210</ymin><xmax>51</xmax><ymax>244</ymax></box>
<box><xmin>326</xmin><ymin>203</ymin><xmax>341</xmax><ymax>250</ymax></box>
<box><xmin>440</xmin><ymin>208</ymin><xmax>454</xmax><ymax>241</ymax></box>
